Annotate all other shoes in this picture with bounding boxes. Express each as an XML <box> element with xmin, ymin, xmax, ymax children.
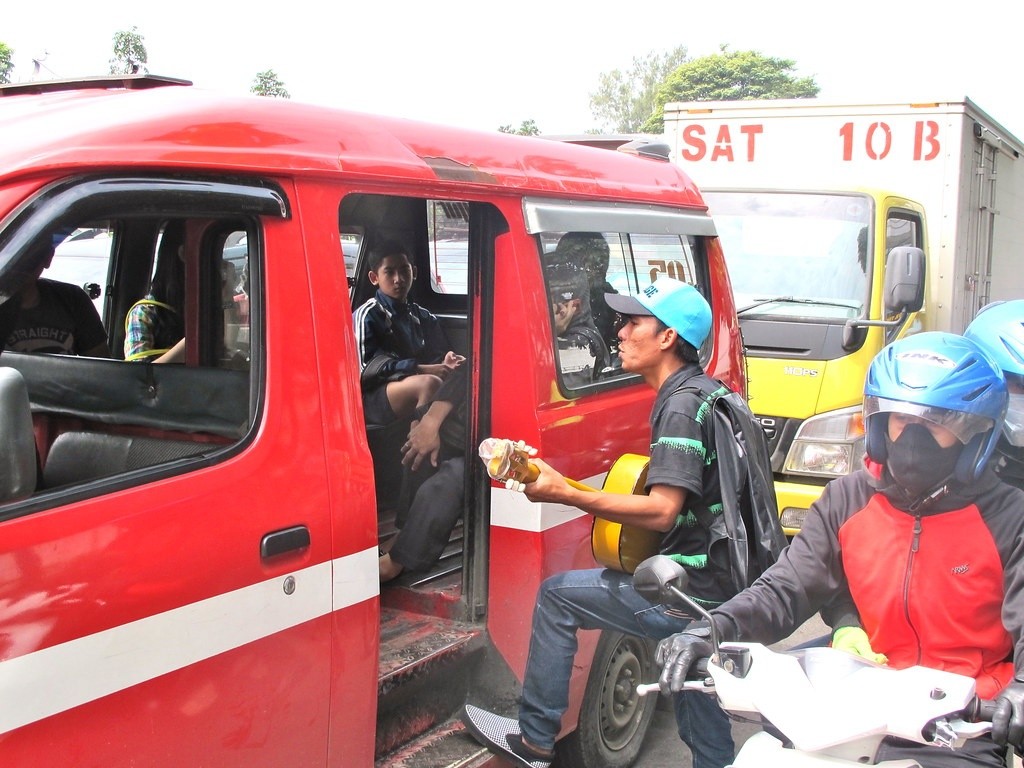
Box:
<box><xmin>461</xmin><ymin>704</ymin><xmax>554</xmax><ymax>768</ymax></box>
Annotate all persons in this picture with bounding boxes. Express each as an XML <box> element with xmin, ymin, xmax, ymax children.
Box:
<box><xmin>353</xmin><ymin>242</ymin><xmax>466</xmax><ymax>528</ymax></box>
<box><xmin>379</xmin><ymin>250</ymin><xmax>611</xmax><ymax>586</ymax></box>
<box><xmin>655</xmin><ymin>330</ymin><xmax>1024</xmax><ymax>768</ymax></box>
<box><xmin>124</xmin><ymin>243</ymin><xmax>250</xmax><ymax>364</ymax></box>
<box><xmin>555</xmin><ymin>231</ymin><xmax>628</xmax><ymax>369</ymax></box>
<box><xmin>819</xmin><ymin>297</ymin><xmax>1024</xmax><ymax>666</ymax></box>
<box><xmin>0</xmin><ymin>230</ymin><xmax>113</xmax><ymax>360</ymax></box>
<box><xmin>459</xmin><ymin>277</ymin><xmax>735</xmax><ymax>768</ymax></box>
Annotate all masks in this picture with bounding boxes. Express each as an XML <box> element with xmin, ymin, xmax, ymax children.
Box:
<box><xmin>884</xmin><ymin>422</ymin><xmax>962</xmax><ymax>506</ymax></box>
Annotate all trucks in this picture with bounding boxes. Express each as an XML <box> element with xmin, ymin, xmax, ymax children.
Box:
<box><xmin>662</xmin><ymin>102</ymin><xmax>1023</xmax><ymax>545</ymax></box>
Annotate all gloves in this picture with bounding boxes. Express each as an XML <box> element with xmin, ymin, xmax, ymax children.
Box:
<box><xmin>652</xmin><ymin>620</ymin><xmax>732</xmax><ymax>693</ymax></box>
<box><xmin>992</xmin><ymin>673</ymin><xmax>1024</xmax><ymax>747</ymax></box>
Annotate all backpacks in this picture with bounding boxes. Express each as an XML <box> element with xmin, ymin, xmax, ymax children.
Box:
<box><xmin>652</xmin><ymin>386</ymin><xmax>788</xmax><ymax>591</ymax></box>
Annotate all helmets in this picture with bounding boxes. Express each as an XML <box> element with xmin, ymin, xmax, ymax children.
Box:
<box><xmin>965</xmin><ymin>300</ymin><xmax>1024</xmax><ymax>376</ymax></box>
<box><xmin>866</xmin><ymin>332</ymin><xmax>1009</xmax><ymax>483</ymax></box>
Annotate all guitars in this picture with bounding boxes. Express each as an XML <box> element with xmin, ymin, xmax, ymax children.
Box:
<box><xmin>476</xmin><ymin>432</ymin><xmax>662</xmax><ymax>575</ymax></box>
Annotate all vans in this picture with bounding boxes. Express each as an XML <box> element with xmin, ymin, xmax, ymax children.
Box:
<box><xmin>1</xmin><ymin>87</ymin><xmax>742</xmax><ymax>767</ymax></box>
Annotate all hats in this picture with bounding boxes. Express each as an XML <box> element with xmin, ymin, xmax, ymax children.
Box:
<box><xmin>603</xmin><ymin>277</ymin><xmax>712</xmax><ymax>350</ymax></box>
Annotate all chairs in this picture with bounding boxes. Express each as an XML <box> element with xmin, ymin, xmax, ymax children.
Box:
<box><xmin>40</xmin><ymin>426</ymin><xmax>229</xmax><ymax>571</ymax></box>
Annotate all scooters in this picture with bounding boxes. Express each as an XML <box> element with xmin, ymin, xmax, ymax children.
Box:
<box><xmin>628</xmin><ymin>555</ymin><xmax>1024</xmax><ymax>768</ymax></box>
